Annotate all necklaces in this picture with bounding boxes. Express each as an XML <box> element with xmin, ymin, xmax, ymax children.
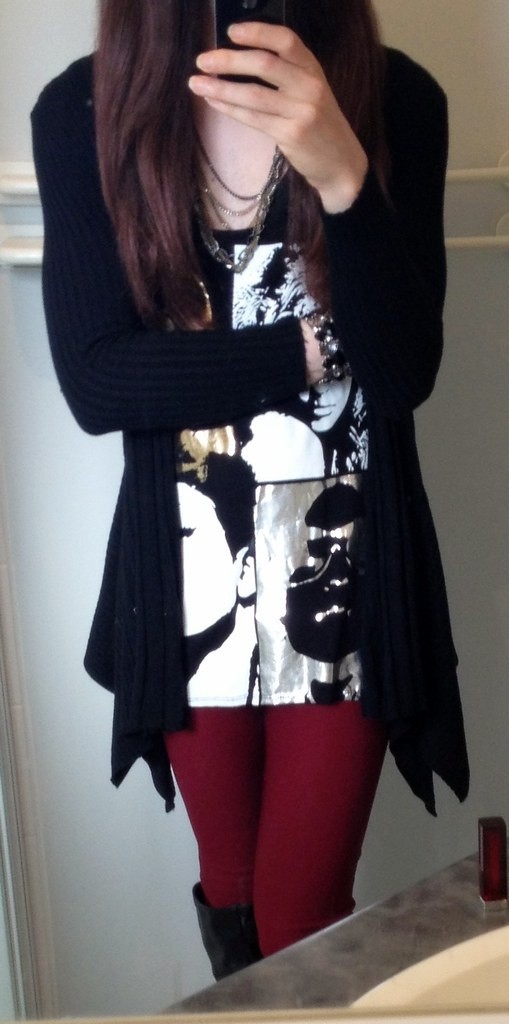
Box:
<box><xmin>192</xmin><ymin>124</ymin><xmax>284</xmax><ymax>275</ymax></box>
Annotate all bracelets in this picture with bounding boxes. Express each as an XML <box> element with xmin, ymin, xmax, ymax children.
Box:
<box><xmin>301</xmin><ymin>299</ymin><xmax>349</xmax><ymax>384</ymax></box>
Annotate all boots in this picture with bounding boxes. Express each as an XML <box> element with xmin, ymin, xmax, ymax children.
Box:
<box><xmin>193</xmin><ymin>882</ymin><xmax>260</xmax><ymax>981</ymax></box>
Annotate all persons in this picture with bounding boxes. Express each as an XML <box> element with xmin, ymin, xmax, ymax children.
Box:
<box><xmin>34</xmin><ymin>0</ymin><xmax>473</xmax><ymax>982</ymax></box>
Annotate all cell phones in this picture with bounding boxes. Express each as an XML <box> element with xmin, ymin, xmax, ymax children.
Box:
<box><xmin>214</xmin><ymin>0</ymin><xmax>290</xmax><ymax>95</ymax></box>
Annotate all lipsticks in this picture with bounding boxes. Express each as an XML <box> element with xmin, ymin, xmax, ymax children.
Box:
<box><xmin>477</xmin><ymin>816</ymin><xmax>509</xmax><ymax>911</ymax></box>
